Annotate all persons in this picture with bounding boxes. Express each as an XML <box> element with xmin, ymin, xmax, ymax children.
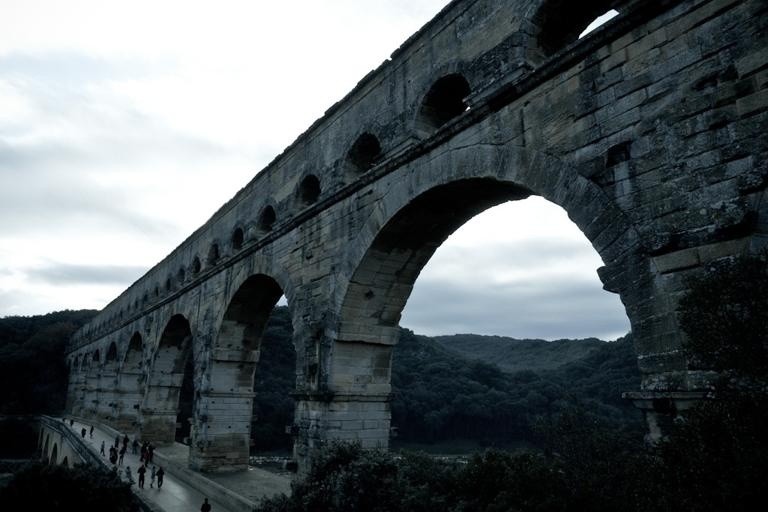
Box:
<box><xmin>61</xmin><ymin>417</ymin><xmax>211</xmax><ymax>512</ymax></box>
